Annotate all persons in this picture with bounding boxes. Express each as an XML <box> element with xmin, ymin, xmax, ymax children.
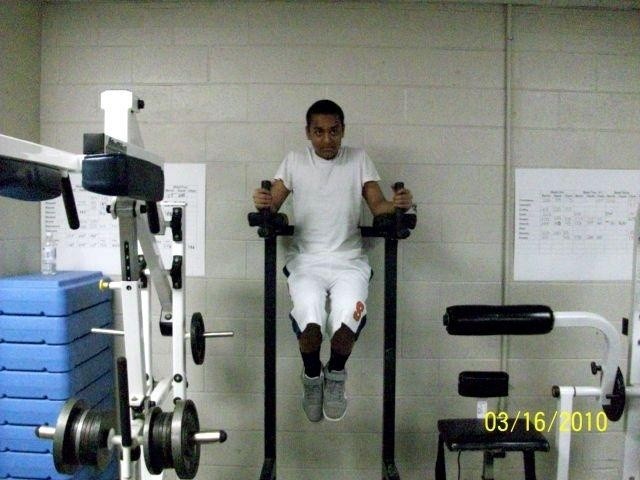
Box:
<box><xmin>252</xmin><ymin>99</ymin><xmax>412</xmax><ymax>425</ymax></box>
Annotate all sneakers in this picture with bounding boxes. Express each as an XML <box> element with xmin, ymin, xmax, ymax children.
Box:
<box><xmin>301</xmin><ymin>361</ymin><xmax>325</xmax><ymax>422</ymax></box>
<box><xmin>322</xmin><ymin>361</ymin><xmax>347</xmax><ymax>421</ymax></box>
